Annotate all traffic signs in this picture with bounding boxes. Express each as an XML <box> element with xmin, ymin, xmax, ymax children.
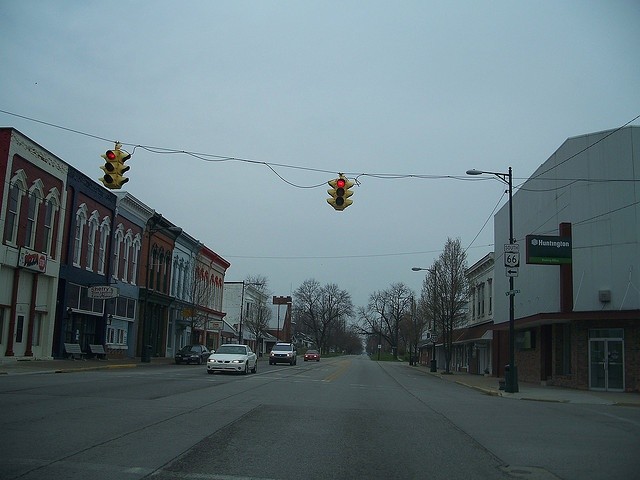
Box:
<box><xmin>504</xmin><ymin>244</ymin><xmax>519</xmax><ymax>277</ymax></box>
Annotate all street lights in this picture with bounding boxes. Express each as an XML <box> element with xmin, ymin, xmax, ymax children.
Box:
<box><xmin>239</xmin><ymin>279</ymin><xmax>262</xmax><ymax>344</ymax></box>
<box><xmin>277</xmin><ymin>301</ymin><xmax>293</xmax><ymax>341</ymax></box>
<box><xmin>141</xmin><ymin>225</ymin><xmax>183</xmax><ymax>363</ymax></box>
<box><xmin>466</xmin><ymin>167</ymin><xmax>519</xmax><ymax>393</ymax></box>
<box><xmin>398</xmin><ymin>296</ymin><xmax>413</xmax><ymax>366</ymax></box>
<box><xmin>412</xmin><ymin>266</ymin><xmax>437</xmax><ymax>372</ymax></box>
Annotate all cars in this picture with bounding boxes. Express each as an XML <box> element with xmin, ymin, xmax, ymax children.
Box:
<box><xmin>175</xmin><ymin>345</ymin><xmax>212</xmax><ymax>364</ymax></box>
<box><xmin>304</xmin><ymin>350</ymin><xmax>320</xmax><ymax>362</ymax></box>
<box><xmin>206</xmin><ymin>344</ymin><xmax>258</xmax><ymax>374</ymax></box>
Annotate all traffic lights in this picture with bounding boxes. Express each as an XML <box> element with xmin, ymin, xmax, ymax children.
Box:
<box><xmin>346</xmin><ymin>179</ymin><xmax>354</xmax><ymax>208</ymax></box>
<box><xmin>326</xmin><ymin>179</ymin><xmax>335</xmax><ymax>208</ymax></box>
<box><xmin>99</xmin><ymin>151</ymin><xmax>106</xmax><ymax>186</ymax></box>
<box><xmin>335</xmin><ymin>177</ymin><xmax>346</xmax><ymax>209</ymax></box>
<box><xmin>118</xmin><ymin>150</ymin><xmax>131</xmax><ymax>188</ymax></box>
<box><xmin>104</xmin><ymin>150</ymin><xmax>118</xmax><ymax>186</ymax></box>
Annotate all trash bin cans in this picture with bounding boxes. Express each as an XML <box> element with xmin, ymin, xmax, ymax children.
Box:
<box><xmin>505</xmin><ymin>363</ymin><xmax>518</xmax><ymax>392</ymax></box>
<box><xmin>408</xmin><ymin>357</ymin><xmax>413</xmax><ymax>365</ymax></box>
<box><xmin>430</xmin><ymin>359</ymin><xmax>437</xmax><ymax>372</ymax></box>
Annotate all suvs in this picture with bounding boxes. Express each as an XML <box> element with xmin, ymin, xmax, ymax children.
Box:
<box><xmin>269</xmin><ymin>341</ymin><xmax>297</xmax><ymax>366</ymax></box>
